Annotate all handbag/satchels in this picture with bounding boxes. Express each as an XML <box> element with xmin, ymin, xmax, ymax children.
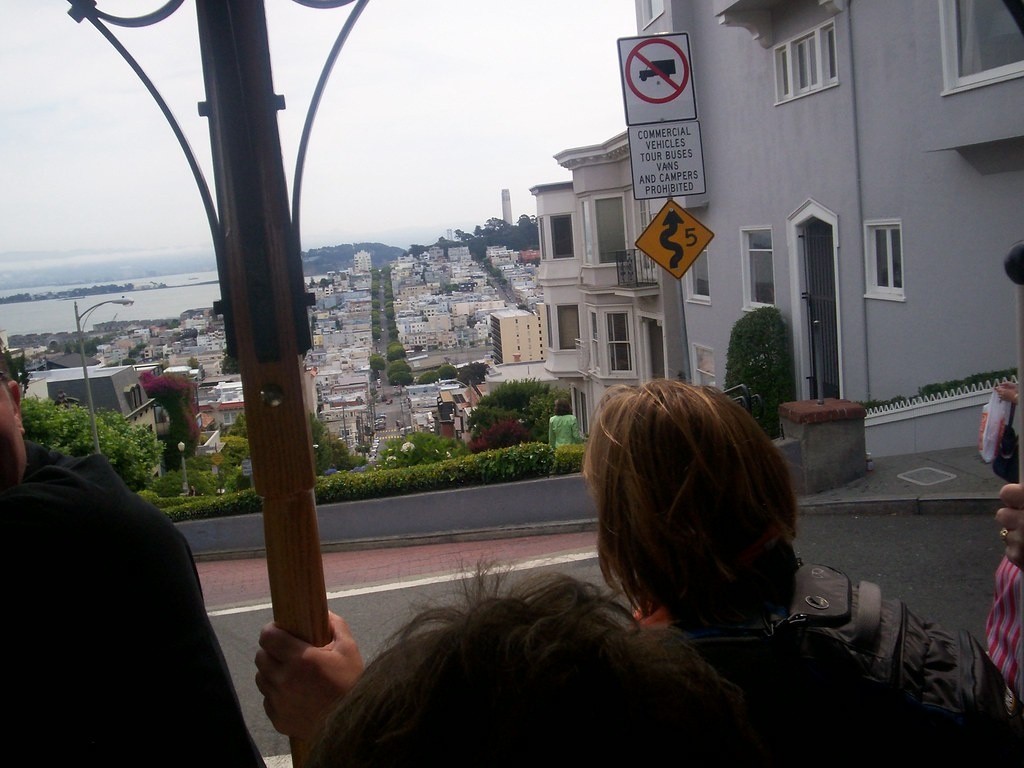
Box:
<box><xmin>978</xmin><ymin>389</ymin><xmax>1008</xmax><ymax>464</ymax></box>
<box><xmin>993</xmin><ymin>402</ymin><xmax>1020</xmax><ymax>484</ymax></box>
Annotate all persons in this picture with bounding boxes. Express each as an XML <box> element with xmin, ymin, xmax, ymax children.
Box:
<box><xmin>0</xmin><ymin>355</ymin><xmax>268</xmax><ymax>768</ymax></box>
<box><xmin>995</xmin><ymin>482</ymin><xmax>1024</xmax><ymax>573</ymax></box>
<box><xmin>255</xmin><ymin>380</ymin><xmax>1024</xmax><ymax>768</ymax></box>
<box><xmin>994</xmin><ymin>381</ymin><xmax>1020</xmax><ymax>405</ymax></box>
<box><xmin>304</xmin><ymin>554</ymin><xmax>770</xmax><ymax>768</ymax></box>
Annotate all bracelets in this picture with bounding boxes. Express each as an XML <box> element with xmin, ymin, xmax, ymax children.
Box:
<box><xmin>1011</xmin><ymin>394</ymin><xmax>1018</xmax><ymax>402</ymax></box>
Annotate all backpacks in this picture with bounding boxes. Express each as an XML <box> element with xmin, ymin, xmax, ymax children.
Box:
<box><xmin>687</xmin><ymin>564</ymin><xmax>1024</xmax><ymax>768</ymax></box>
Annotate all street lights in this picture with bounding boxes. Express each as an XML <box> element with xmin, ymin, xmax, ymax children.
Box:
<box><xmin>73</xmin><ymin>296</ymin><xmax>135</xmax><ymax>456</ymax></box>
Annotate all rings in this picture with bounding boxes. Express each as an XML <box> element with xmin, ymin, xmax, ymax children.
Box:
<box><xmin>999</xmin><ymin>529</ymin><xmax>1009</xmax><ymax>540</ymax></box>
